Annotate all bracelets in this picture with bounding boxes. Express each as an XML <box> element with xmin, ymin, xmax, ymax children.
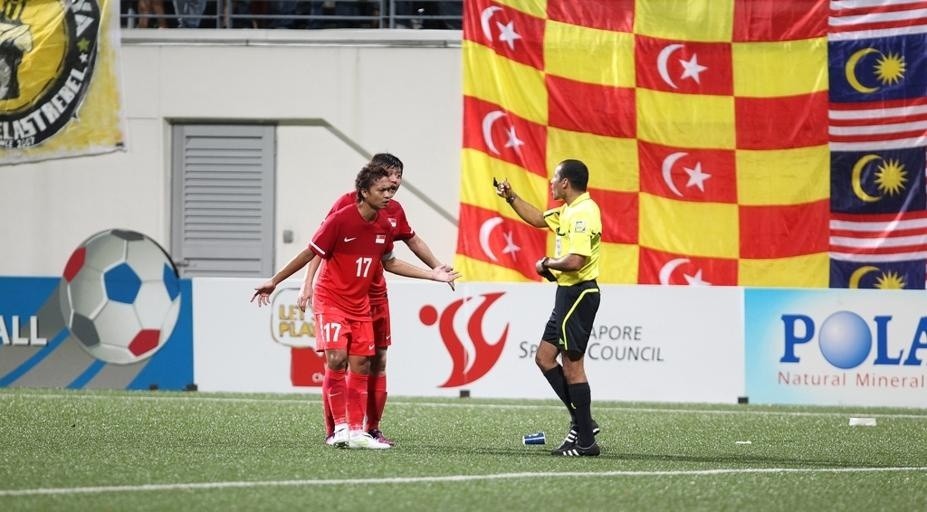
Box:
<box><xmin>505</xmin><ymin>192</ymin><xmax>517</xmax><ymax>205</ymax></box>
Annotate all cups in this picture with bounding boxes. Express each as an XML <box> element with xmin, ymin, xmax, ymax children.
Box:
<box><xmin>521</xmin><ymin>431</ymin><xmax>546</xmax><ymax>445</ymax></box>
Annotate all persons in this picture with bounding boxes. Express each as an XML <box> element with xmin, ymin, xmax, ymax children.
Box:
<box><xmin>249</xmin><ymin>151</ymin><xmax>456</xmax><ymax>447</ymax></box>
<box><xmin>492</xmin><ymin>157</ymin><xmax>604</xmax><ymax>458</ymax></box>
<box><xmin>120</xmin><ymin>0</ymin><xmax>462</xmax><ymax>34</ymax></box>
<box><xmin>292</xmin><ymin>163</ymin><xmax>464</xmax><ymax>451</ymax></box>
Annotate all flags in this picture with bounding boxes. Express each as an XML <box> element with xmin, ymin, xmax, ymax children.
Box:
<box><xmin>0</xmin><ymin>0</ymin><xmax>129</xmax><ymax>169</ymax></box>
<box><xmin>450</xmin><ymin>1</ymin><xmax>927</xmax><ymax>293</ymax></box>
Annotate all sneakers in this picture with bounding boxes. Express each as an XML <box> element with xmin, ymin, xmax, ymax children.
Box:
<box><xmin>555</xmin><ymin>419</ymin><xmax>599</xmax><ymax>448</ymax></box>
<box><xmin>324</xmin><ymin>426</ymin><xmax>393</xmax><ymax>449</ymax></box>
<box><xmin>556</xmin><ymin>440</ymin><xmax>599</xmax><ymax>456</ymax></box>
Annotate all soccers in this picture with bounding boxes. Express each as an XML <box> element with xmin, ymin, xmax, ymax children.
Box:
<box><xmin>58</xmin><ymin>228</ymin><xmax>181</xmax><ymax>364</ymax></box>
